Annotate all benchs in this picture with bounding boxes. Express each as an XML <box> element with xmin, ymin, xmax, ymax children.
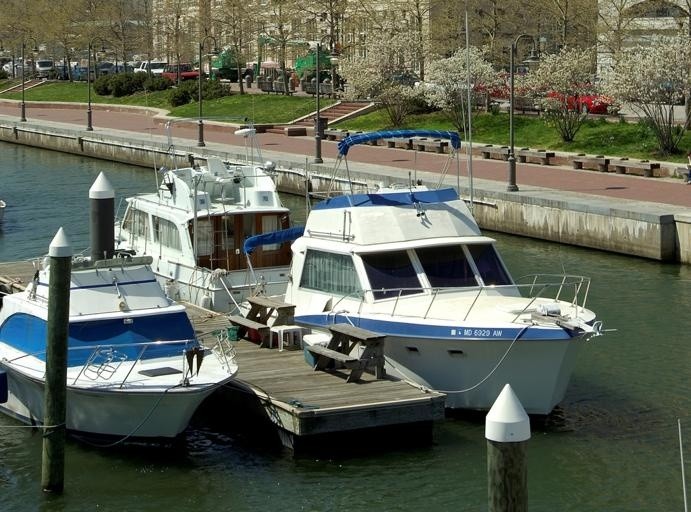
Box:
<box><xmin>305</xmin><ymin>344</ymin><xmax>365</xmax><ymax>384</ymax></box>
<box><xmin>225</xmin><ymin>314</ymin><xmax>270</xmax><ymax>349</ymax></box>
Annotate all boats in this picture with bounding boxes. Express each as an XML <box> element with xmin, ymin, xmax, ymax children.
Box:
<box><xmin>109</xmin><ymin>120</ymin><xmax>298</xmax><ymax>318</ymax></box>
<box><xmin>0</xmin><ymin>251</ymin><xmax>237</xmax><ymax>455</ymax></box>
<box><xmin>237</xmin><ymin>128</ymin><xmax>618</xmax><ymax>428</ymax></box>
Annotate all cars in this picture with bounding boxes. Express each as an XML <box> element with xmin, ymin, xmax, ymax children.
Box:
<box><xmin>4</xmin><ymin>54</ymin><xmax>252</xmax><ymax>83</ymax></box>
<box><xmin>370</xmin><ymin>67</ymin><xmax>618</xmax><ymax>115</ymax></box>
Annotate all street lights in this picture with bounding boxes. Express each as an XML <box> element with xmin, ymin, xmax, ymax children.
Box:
<box><xmin>312</xmin><ymin>34</ymin><xmax>340</xmax><ymax>164</ymax></box>
<box><xmin>20</xmin><ymin>38</ymin><xmax>40</xmax><ymax>123</ymax></box>
<box><xmin>86</xmin><ymin>36</ymin><xmax>108</xmax><ymax>130</ymax></box>
<box><xmin>328</xmin><ymin>41</ymin><xmax>339</xmax><ymax>98</ymax></box>
<box><xmin>196</xmin><ymin>35</ymin><xmax>220</xmax><ymax>147</ymax></box>
<box><xmin>507</xmin><ymin>34</ymin><xmax>539</xmax><ymax>190</ymax></box>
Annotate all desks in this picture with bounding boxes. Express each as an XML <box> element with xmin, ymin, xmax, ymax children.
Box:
<box><xmin>234</xmin><ymin>296</ymin><xmax>299</xmax><ymax>348</ymax></box>
<box><xmin>314</xmin><ymin>322</ymin><xmax>391</xmax><ymax>383</ymax></box>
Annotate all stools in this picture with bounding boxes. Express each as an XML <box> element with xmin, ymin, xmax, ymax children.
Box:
<box><xmin>272</xmin><ymin>323</ymin><xmax>303</xmax><ymax>352</ymax></box>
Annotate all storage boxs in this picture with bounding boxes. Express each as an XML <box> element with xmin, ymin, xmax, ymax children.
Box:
<box><xmin>303</xmin><ymin>331</ymin><xmax>330</xmax><ymax>367</ymax></box>
